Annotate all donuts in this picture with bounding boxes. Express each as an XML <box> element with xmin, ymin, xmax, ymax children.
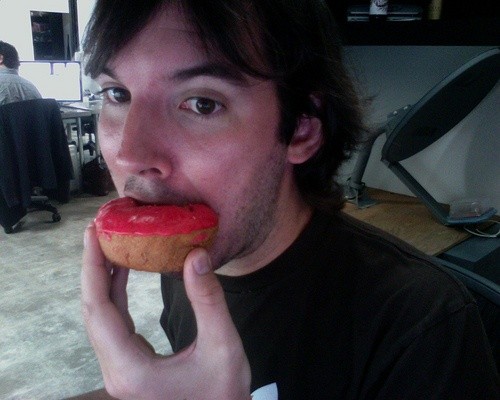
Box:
<box><xmin>96</xmin><ymin>196</ymin><xmax>219</xmax><ymax>274</ymax></box>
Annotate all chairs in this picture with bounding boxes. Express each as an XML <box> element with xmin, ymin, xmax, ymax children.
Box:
<box><xmin>2</xmin><ymin>98</ymin><xmax>75</xmax><ymax>235</ymax></box>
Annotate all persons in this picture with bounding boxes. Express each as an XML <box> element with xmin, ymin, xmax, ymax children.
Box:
<box><xmin>0</xmin><ymin>40</ymin><xmax>43</xmax><ymax>106</ymax></box>
<box><xmin>79</xmin><ymin>0</ymin><xmax>500</xmax><ymax>400</ymax></box>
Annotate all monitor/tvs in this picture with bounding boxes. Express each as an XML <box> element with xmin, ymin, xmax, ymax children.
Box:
<box><xmin>18</xmin><ymin>61</ymin><xmax>83</xmax><ymax>112</ymax></box>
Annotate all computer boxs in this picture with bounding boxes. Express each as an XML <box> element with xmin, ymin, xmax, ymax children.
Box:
<box><xmin>67</xmin><ymin>144</ymin><xmax>83</xmax><ymax>193</ymax></box>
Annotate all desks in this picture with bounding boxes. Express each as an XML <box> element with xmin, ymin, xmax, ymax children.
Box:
<box><xmin>69</xmin><ymin>98</ymin><xmax>105</xmax><ymax>163</ymax></box>
<box><xmin>60</xmin><ymin>106</ymin><xmax>94</xmax><ymax>168</ymax></box>
<box><xmin>64</xmin><ymin>92</ymin><xmax>103</xmax><ymax>166</ymax></box>
<box><xmin>334</xmin><ymin>176</ymin><xmax>483</xmax><ymax>259</ymax></box>
